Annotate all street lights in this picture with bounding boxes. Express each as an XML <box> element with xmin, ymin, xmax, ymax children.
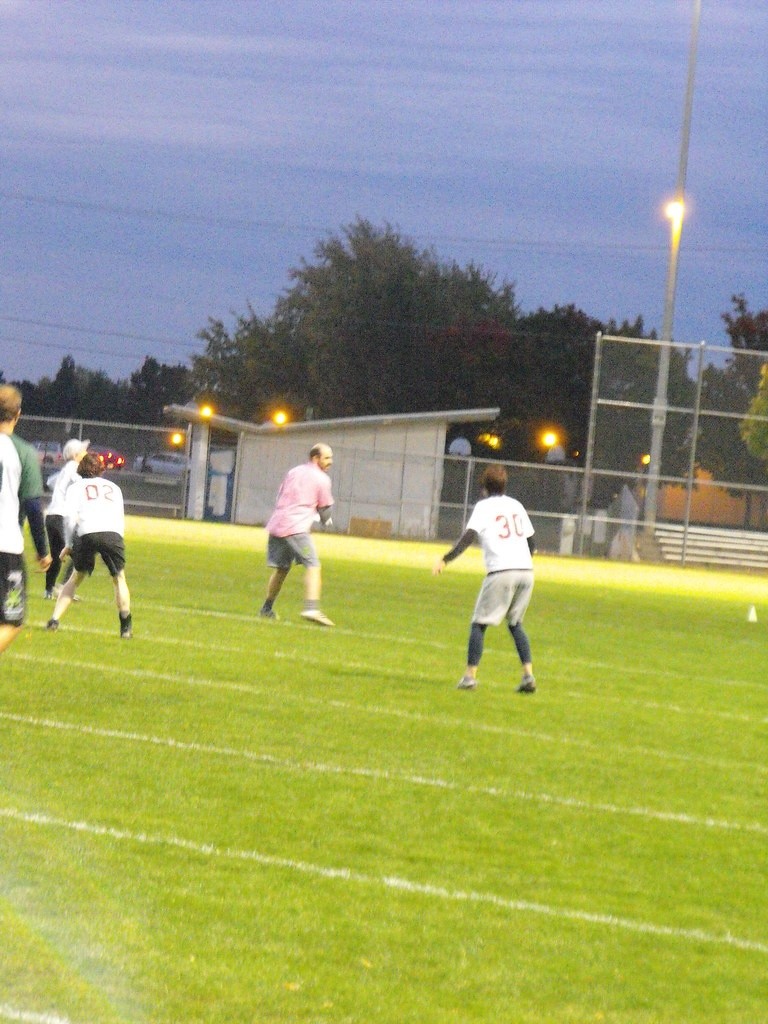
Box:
<box><xmin>635</xmin><ymin>197</ymin><xmax>688</xmax><ymax>564</ymax></box>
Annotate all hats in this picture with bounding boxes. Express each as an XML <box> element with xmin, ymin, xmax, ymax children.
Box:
<box><xmin>62</xmin><ymin>435</ymin><xmax>89</xmax><ymax>461</ymax></box>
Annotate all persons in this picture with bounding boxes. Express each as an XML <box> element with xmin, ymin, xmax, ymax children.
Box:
<box><xmin>0</xmin><ymin>383</ymin><xmax>52</xmax><ymax>652</ymax></box>
<box><xmin>43</xmin><ymin>437</ymin><xmax>90</xmax><ymax>602</ymax></box>
<box><xmin>433</xmin><ymin>466</ymin><xmax>539</xmax><ymax>697</ymax></box>
<box><xmin>259</xmin><ymin>443</ymin><xmax>337</xmax><ymax>627</ymax></box>
<box><xmin>47</xmin><ymin>452</ymin><xmax>133</xmax><ymax>639</ymax></box>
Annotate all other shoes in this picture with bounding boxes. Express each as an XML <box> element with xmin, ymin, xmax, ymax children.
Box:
<box><xmin>457</xmin><ymin>678</ymin><xmax>477</xmax><ymax>688</ymax></box>
<box><xmin>47</xmin><ymin>621</ymin><xmax>58</xmax><ymax>633</ymax></box>
<box><xmin>516</xmin><ymin>675</ymin><xmax>537</xmax><ymax>693</ymax></box>
<box><xmin>260</xmin><ymin>610</ymin><xmax>280</xmax><ymax>621</ymax></box>
<box><xmin>58</xmin><ymin>583</ymin><xmax>79</xmax><ymax>601</ymax></box>
<box><xmin>119</xmin><ymin>627</ymin><xmax>135</xmax><ymax>638</ymax></box>
<box><xmin>303</xmin><ymin>609</ymin><xmax>333</xmax><ymax>626</ymax></box>
<box><xmin>44</xmin><ymin>590</ymin><xmax>57</xmax><ymax>600</ymax></box>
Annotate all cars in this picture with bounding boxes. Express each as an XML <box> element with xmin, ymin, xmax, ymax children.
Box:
<box><xmin>132</xmin><ymin>451</ymin><xmax>192</xmax><ymax>479</ymax></box>
<box><xmin>32</xmin><ymin>440</ymin><xmax>62</xmax><ymax>466</ymax></box>
<box><xmin>86</xmin><ymin>446</ymin><xmax>127</xmax><ymax>472</ymax></box>
